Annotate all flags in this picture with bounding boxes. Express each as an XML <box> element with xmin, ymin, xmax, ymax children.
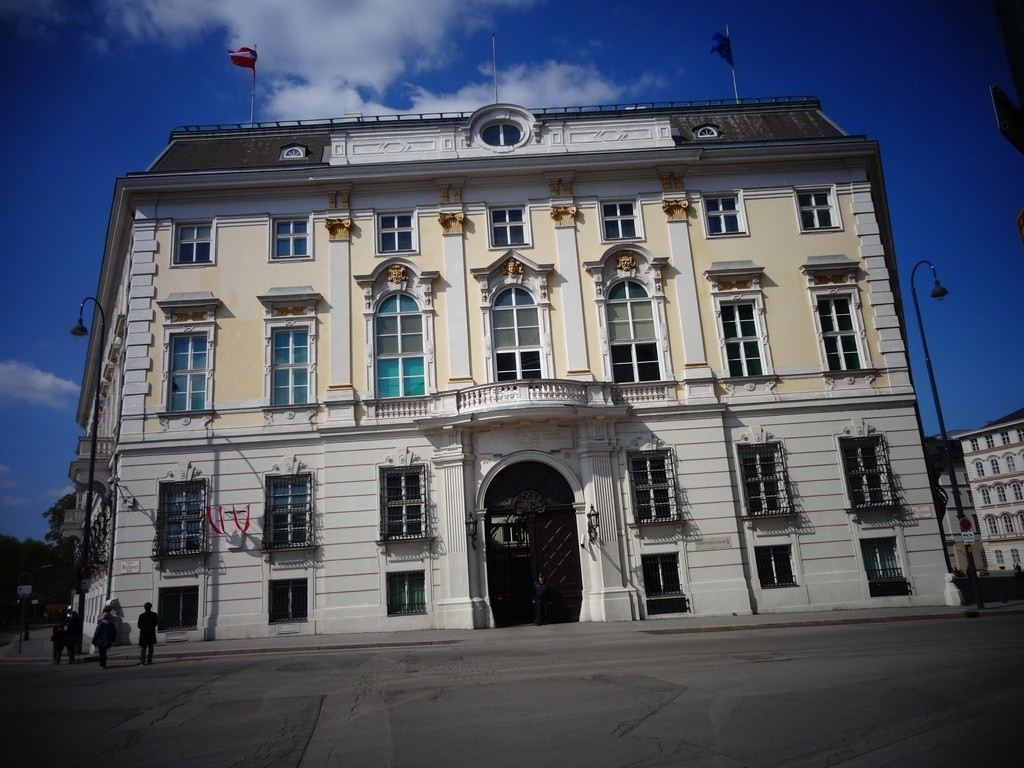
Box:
<box><xmin>244</xmin><ymin>505</ymin><xmax>250</xmax><ymax>532</ymax></box>
<box><xmin>710</xmin><ymin>32</ymin><xmax>734</xmax><ymax>67</ymax></box>
<box><xmin>207</xmin><ymin>505</ymin><xmax>222</xmax><ymax>534</ymax></box>
<box><xmin>227</xmin><ymin>47</ymin><xmax>257</xmax><ymax>73</ymax></box>
<box><xmin>217</xmin><ymin>504</ymin><xmax>227</xmax><ymax>534</ymax></box>
<box><xmin>232</xmin><ymin>505</ymin><xmax>244</xmax><ymax>532</ymax></box>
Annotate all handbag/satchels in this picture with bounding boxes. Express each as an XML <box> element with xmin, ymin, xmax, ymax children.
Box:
<box><xmin>90</xmin><ymin>643</ymin><xmax>95</xmax><ymax>655</ymax></box>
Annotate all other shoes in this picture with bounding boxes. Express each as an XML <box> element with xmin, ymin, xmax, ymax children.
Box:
<box><xmin>138</xmin><ymin>663</ymin><xmax>144</xmax><ymax>666</ymax></box>
<box><xmin>57</xmin><ymin>662</ymin><xmax>60</xmax><ymax>664</ymax></box>
<box><xmin>542</xmin><ymin>621</ymin><xmax>547</xmax><ymax>625</ymax></box>
<box><xmin>147</xmin><ymin>661</ymin><xmax>152</xmax><ymax>664</ymax></box>
<box><xmin>69</xmin><ymin>659</ymin><xmax>75</xmax><ymax>664</ymax></box>
<box><xmin>535</xmin><ymin>618</ymin><xmax>541</xmax><ymax>626</ymax></box>
<box><xmin>52</xmin><ymin>660</ymin><xmax>57</xmax><ymax>664</ymax></box>
<box><xmin>99</xmin><ymin>666</ymin><xmax>104</xmax><ymax>669</ymax></box>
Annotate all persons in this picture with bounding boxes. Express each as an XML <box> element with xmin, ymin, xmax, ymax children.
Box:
<box><xmin>91</xmin><ymin>608</ymin><xmax>117</xmax><ymax>670</ymax></box>
<box><xmin>138</xmin><ymin>602</ymin><xmax>158</xmax><ymax>667</ymax></box>
<box><xmin>531</xmin><ymin>571</ymin><xmax>553</xmax><ymax>626</ymax></box>
<box><xmin>51</xmin><ymin>611</ymin><xmax>79</xmax><ymax>665</ymax></box>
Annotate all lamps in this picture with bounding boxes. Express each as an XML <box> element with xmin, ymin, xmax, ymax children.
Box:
<box><xmin>465</xmin><ymin>511</ymin><xmax>478</xmax><ymax>551</ymax></box>
<box><xmin>586</xmin><ymin>503</ymin><xmax>600</xmax><ymax>545</ymax></box>
